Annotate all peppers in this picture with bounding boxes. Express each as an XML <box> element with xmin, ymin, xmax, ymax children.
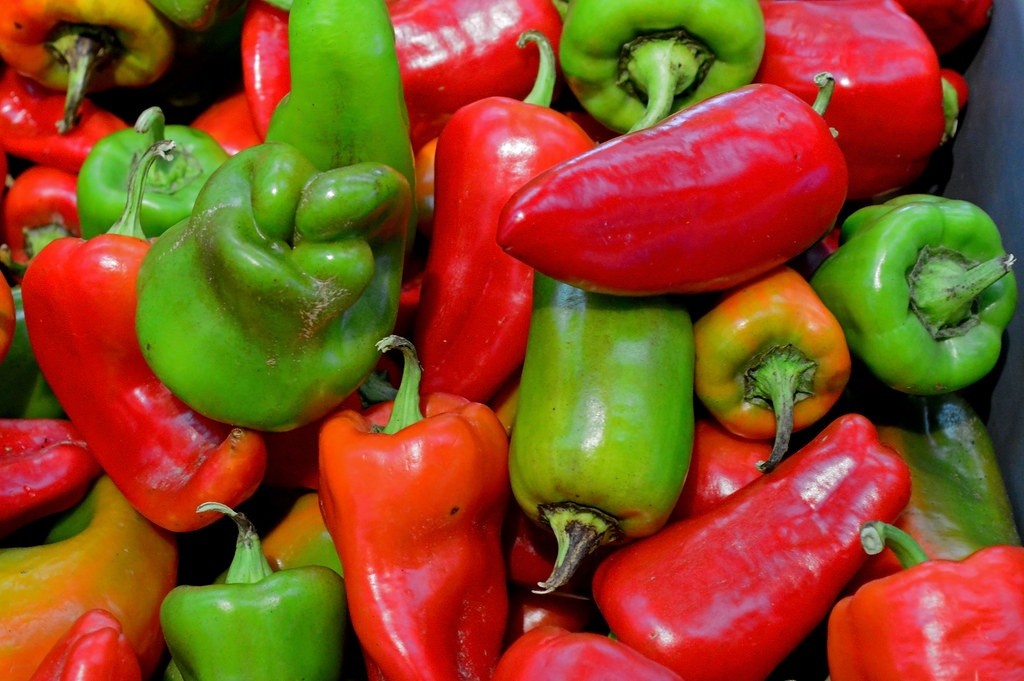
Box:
<box><xmin>0</xmin><ymin>0</ymin><xmax>1024</xmax><ymax>681</ymax></box>
